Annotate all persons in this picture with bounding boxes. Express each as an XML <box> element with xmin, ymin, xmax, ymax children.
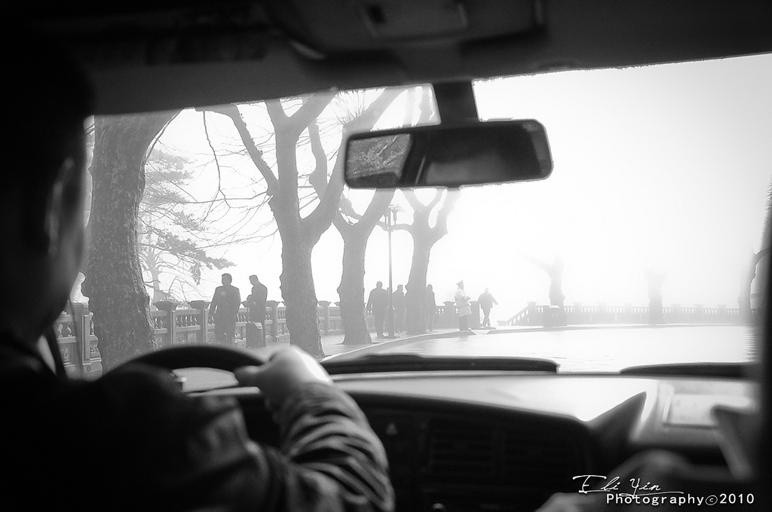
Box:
<box><xmin>241</xmin><ymin>274</ymin><xmax>268</xmax><ymax>347</ymax></box>
<box><xmin>425</xmin><ymin>124</ymin><xmax>542</xmax><ymax>183</ymax></box>
<box><xmin>1</xmin><ymin>1</ymin><xmax>397</xmax><ymax>512</ymax></box>
<box><xmin>207</xmin><ymin>273</ymin><xmax>240</xmax><ymax>347</ymax></box>
<box><xmin>366</xmin><ymin>278</ymin><xmax>498</xmax><ymax>339</ymax></box>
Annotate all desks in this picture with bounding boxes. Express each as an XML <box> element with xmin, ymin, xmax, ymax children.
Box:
<box><xmin>382</xmin><ymin>206</ymin><xmax>400</xmax><ymax>339</ymax></box>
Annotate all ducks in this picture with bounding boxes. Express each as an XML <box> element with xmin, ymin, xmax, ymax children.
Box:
<box><xmin>246</xmin><ymin>308</ymin><xmax>264</xmax><ymax>349</ymax></box>
